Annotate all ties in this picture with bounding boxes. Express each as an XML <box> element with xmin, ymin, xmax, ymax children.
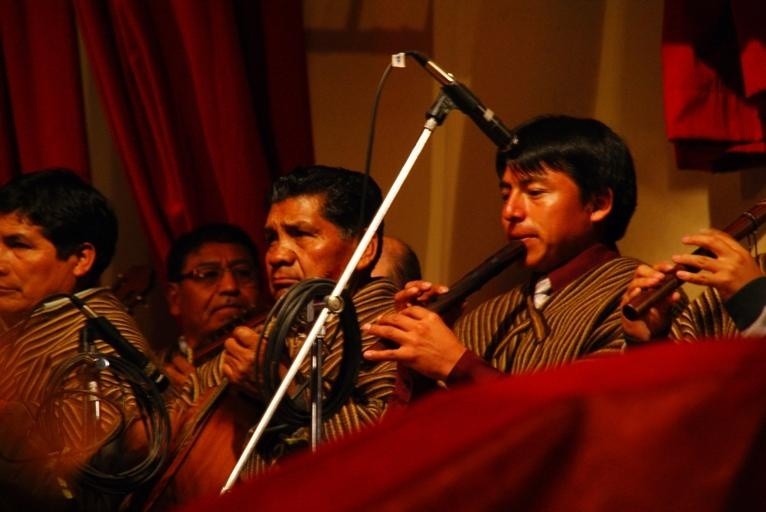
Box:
<box><xmin>532</xmin><ymin>279</ymin><xmax>551</xmax><ymax>309</ymax></box>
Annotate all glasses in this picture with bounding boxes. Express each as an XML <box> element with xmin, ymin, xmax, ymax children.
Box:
<box><xmin>178</xmin><ymin>263</ymin><xmax>256</xmax><ymax>286</ymax></box>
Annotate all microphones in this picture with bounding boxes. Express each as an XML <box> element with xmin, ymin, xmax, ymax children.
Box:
<box><xmin>73</xmin><ymin>296</ymin><xmax>170</xmax><ymax>393</ymax></box>
<box><xmin>416</xmin><ymin>50</ymin><xmax>524</xmax><ymax>160</ymax></box>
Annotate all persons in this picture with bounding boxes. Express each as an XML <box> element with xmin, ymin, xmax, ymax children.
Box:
<box><xmin>174</xmin><ymin>165</ymin><xmax>402</xmax><ymax>483</ymax></box>
<box><xmin>370</xmin><ymin>236</ymin><xmax>421</xmax><ymax>289</ymax></box>
<box><xmin>0</xmin><ymin>167</ymin><xmax>175</xmax><ymax>512</ymax></box>
<box><xmin>164</xmin><ymin>222</ymin><xmax>268</xmax><ymax>341</ymax></box>
<box><xmin>620</xmin><ymin>227</ymin><xmax>766</xmax><ymax>351</ymax></box>
<box><xmin>361</xmin><ymin>114</ymin><xmax>691</xmax><ymax>425</ymax></box>
